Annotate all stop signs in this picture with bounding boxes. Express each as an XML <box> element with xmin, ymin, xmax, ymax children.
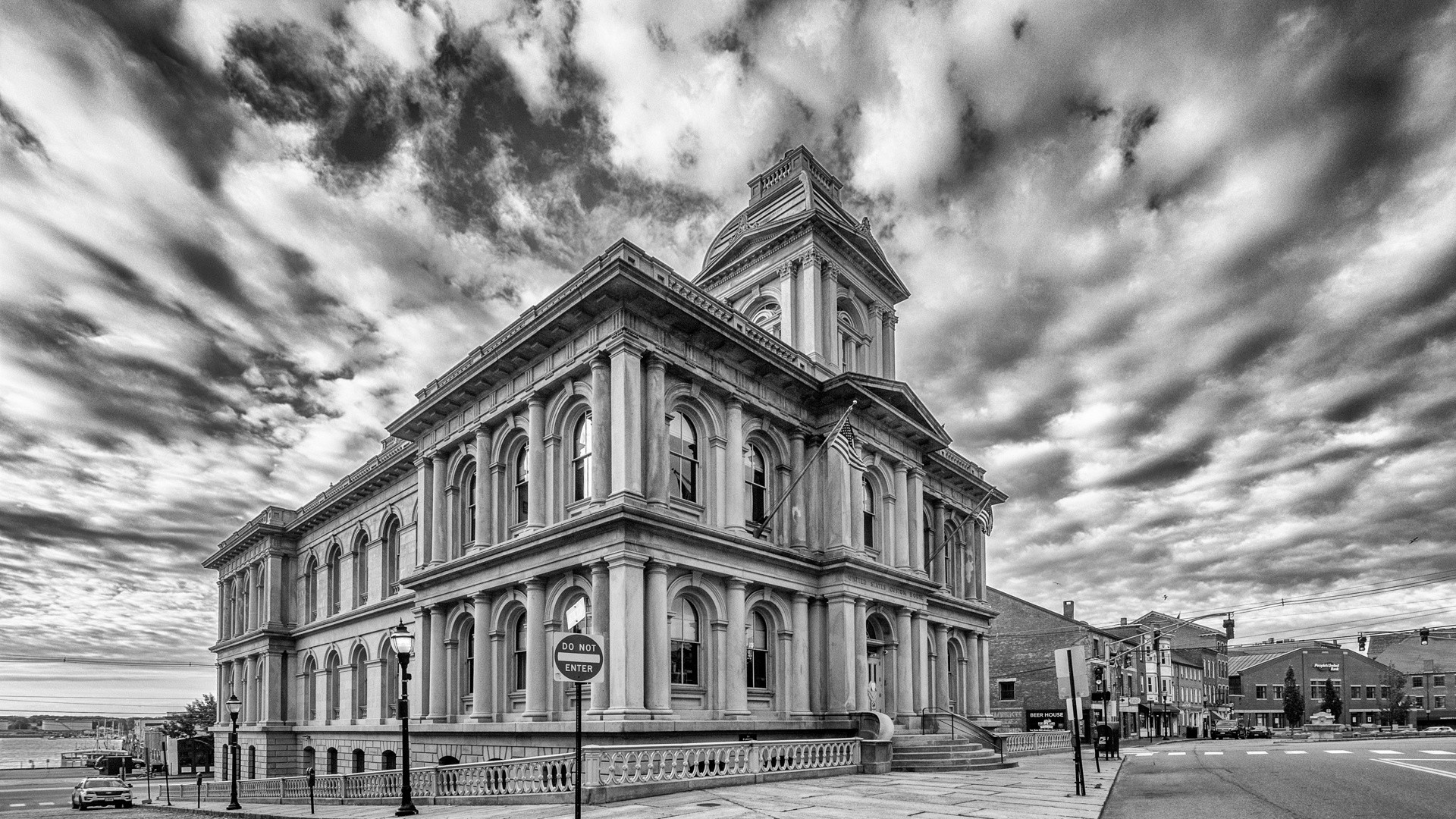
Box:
<box><xmin>554</xmin><ymin>634</ymin><xmax>603</xmax><ymax>681</ymax></box>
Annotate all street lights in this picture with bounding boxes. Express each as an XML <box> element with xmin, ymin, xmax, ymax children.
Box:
<box><xmin>1201</xmin><ymin>693</ymin><xmax>1209</xmax><ymax>738</ymax></box>
<box><xmin>388</xmin><ymin>616</ymin><xmax>419</xmax><ymax>816</ymax></box>
<box><xmin>225</xmin><ymin>690</ymin><xmax>243</xmax><ymax>810</ymax></box>
<box><xmin>1426</xmin><ymin>707</ymin><xmax>1431</xmax><ymax>727</ymax></box>
<box><xmin>1160</xmin><ymin>687</ymin><xmax>1170</xmax><ymax>740</ymax></box>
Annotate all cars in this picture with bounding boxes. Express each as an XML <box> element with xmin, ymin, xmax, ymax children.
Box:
<box><xmin>94</xmin><ymin>754</ymin><xmax>146</xmax><ymax>771</ymax></box>
<box><xmin>1246</xmin><ymin>725</ymin><xmax>1272</xmax><ymax>739</ymax></box>
<box><xmin>1397</xmin><ymin>726</ymin><xmax>1456</xmax><ymax>737</ymax></box>
<box><xmin>72</xmin><ymin>776</ymin><xmax>133</xmax><ymax>811</ymax></box>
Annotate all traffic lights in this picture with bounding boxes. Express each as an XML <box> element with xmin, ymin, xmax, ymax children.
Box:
<box><xmin>1358</xmin><ymin>637</ymin><xmax>1367</xmax><ymax>651</ymax></box>
<box><xmin>1420</xmin><ymin>628</ymin><xmax>1429</xmax><ymax>646</ymax></box>
<box><xmin>1093</xmin><ymin>666</ymin><xmax>1104</xmax><ymax>693</ymax></box>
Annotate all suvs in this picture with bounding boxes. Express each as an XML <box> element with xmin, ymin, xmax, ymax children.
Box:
<box><xmin>1210</xmin><ymin>718</ymin><xmax>1247</xmax><ymax>740</ymax></box>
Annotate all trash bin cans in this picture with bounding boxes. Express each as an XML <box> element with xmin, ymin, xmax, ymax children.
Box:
<box><xmin>152</xmin><ymin>764</ymin><xmax>169</xmax><ymax>776</ymax></box>
<box><xmin>1095</xmin><ymin>725</ymin><xmax>1114</xmax><ymax>758</ymax></box>
<box><xmin>1097</xmin><ymin>721</ymin><xmax>1119</xmax><ymax>757</ymax></box>
<box><xmin>1185</xmin><ymin>727</ymin><xmax>1199</xmax><ymax>739</ymax></box>
